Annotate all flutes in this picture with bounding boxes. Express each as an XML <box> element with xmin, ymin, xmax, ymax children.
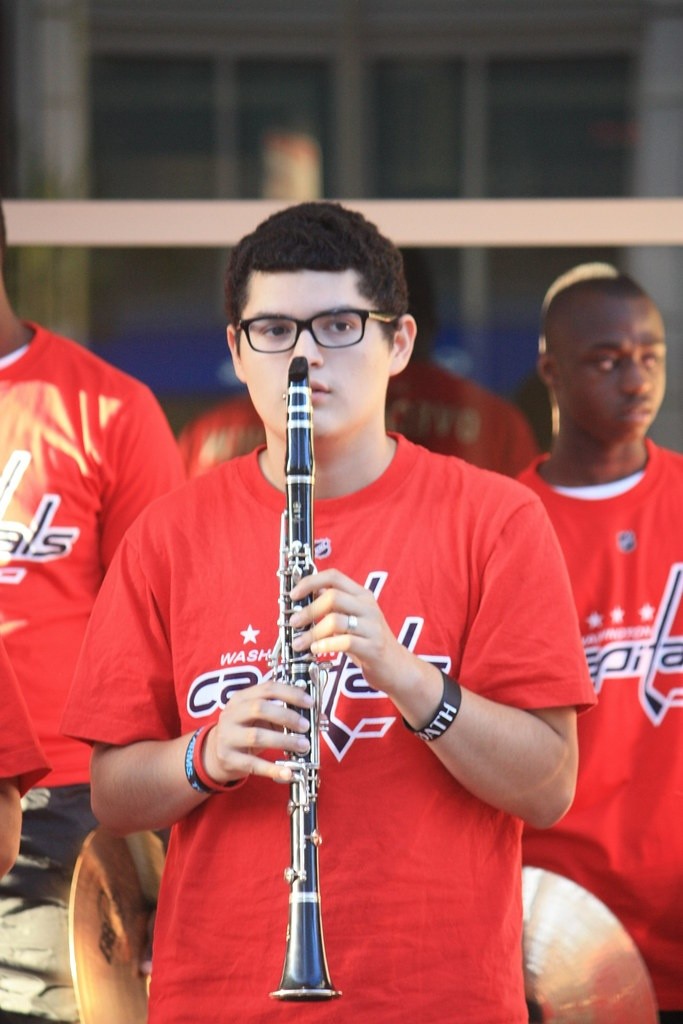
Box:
<box><xmin>266</xmin><ymin>355</ymin><xmax>345</xmax><ymax>1001</ymax></box>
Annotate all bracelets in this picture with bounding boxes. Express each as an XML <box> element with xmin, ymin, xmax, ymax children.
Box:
<box><xmin>402</xmin><ymin>666</ymin><xmax>463</xmax><ymax>740</ymax></box>
<box><xmin>194</xmin><ymin>719</ymin><xmax>250</xmax><ymax>792</ymax></box>
<box><xmin>183</xmin><ymin>727</ymin><xmax>238</xmax><ymax>800</ymax></box>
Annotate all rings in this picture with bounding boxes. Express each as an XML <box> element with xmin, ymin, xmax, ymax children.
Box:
<box><xmin>345</xmin><ymin>614</ymin><xmax>356</xmax><ymax>635</ymax></box>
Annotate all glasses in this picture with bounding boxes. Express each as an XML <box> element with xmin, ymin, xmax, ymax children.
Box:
<box><xmin>234</xmin><ymin>309</ymin><xmax>397</xmax><ymax>354</ymax></box>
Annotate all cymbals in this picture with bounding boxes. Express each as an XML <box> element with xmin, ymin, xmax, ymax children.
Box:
<box><xmin>67</xmin><ymin>824</ymin><xmax>166</xmax><ymax>1024</ymax></box>
<box><xmin>521</xmin><ymin>865</ymin><xmax>656</xmax><ymax>1023</ymax></box>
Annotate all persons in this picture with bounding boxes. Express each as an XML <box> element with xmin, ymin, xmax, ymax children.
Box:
<box><xmin>386</xmin><ymin>249</ymin><xmax>541</xmax><ymax>487</ymax></box>
<box><xmin>0</xmin><ymin>203</ymin><xmax>190</xmax><ymax>1024</ymax></box>
<box><xmin>507</xmin><ymin>258</ymin><xmax>683</xmax><ymax>1024</ymax></box>
<box><xmin>55</xmin><ymin>199</ymin><xmax>600</xmax><ymax>1021</ymax></box>
<box><xmin>170</xmin><ymin>390</ymin><xmax>267</xmax><ymax>483</ymax></box>
